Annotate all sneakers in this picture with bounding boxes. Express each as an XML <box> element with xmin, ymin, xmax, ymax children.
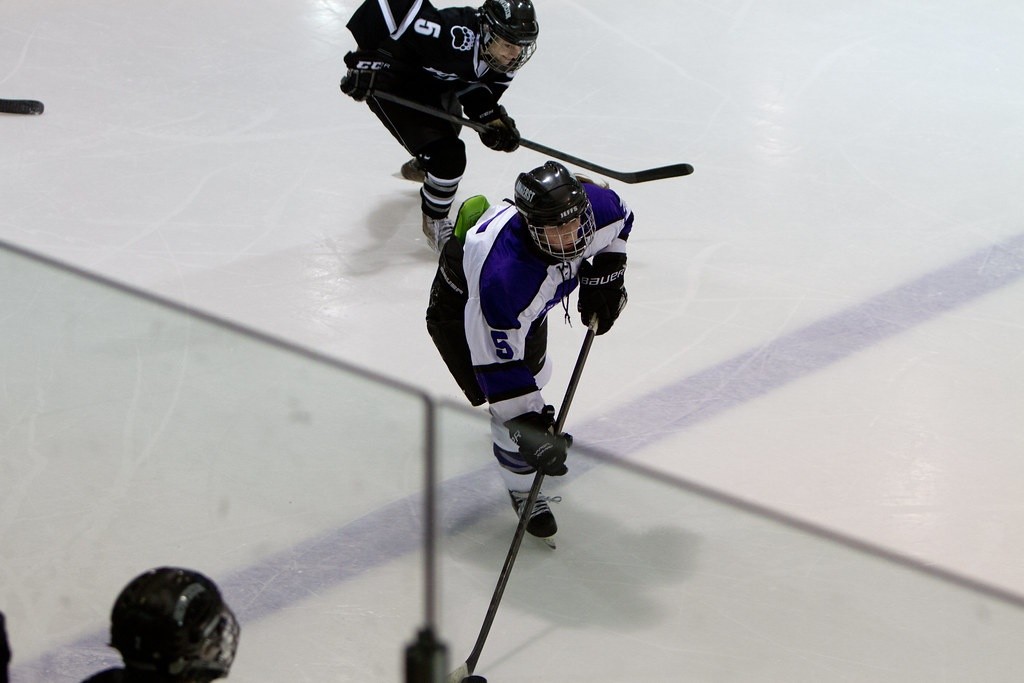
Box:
<box><xmin>401</xmin><ymin>157</ymin><xmax>427</xmax><ymax>183</ymax></box>
<box><xmin>421</xmin><ymin>212</ymin><xmax>454</xmax><ymax>254</ymax></box>
<box><xmin>510</xmin><ymin>489</ymin><xmax>560</xmax><ymax>549</ymax></box>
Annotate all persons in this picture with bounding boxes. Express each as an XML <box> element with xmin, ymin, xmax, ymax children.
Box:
<box><xmin>79</xmin><ymin>566</ymin><xmax>242</xmax><ymax>683</ymax></box>
<box><xmin>425</xmin><ymin>160</ymin><xmax>635</xmax><ymax>549</ymax></box>
<box><xmin>339</xmin><ymin>0</ymin><xmax>539</xmax><ymax>257</ymax></box>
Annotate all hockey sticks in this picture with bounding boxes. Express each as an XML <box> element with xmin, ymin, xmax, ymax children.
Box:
<box><xmin>368</xmin><ymin>86</ymin><xmax>697</xmax><ymax>184</ymax></box>
<box><xmin>442</xmin><ymin>316</ymin><xmax>599</xmax><ymax>683</ymax></box>
<box><xmin>0</xmin><ymin>98</ymin><xmax>47</xmax><ymax>117</ymax></box>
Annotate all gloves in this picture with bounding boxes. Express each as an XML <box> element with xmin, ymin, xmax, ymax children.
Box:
<box><xmin>464</xmin><ymin>103</ymin><xmax>521</xmax><ymax>152</ymax></box>
<box><xmin>502</xmin><ymin>404</ymin><xmax>572</xmax><ymax>477</ymax></box>
<box><xmin>577</xmin><ymin>251</ymin><xmax>628</xmax><ymax>336</ymax></box>
<box><xmin>341</xmin><ymin>50</ymin><xmax>393</xmax><ymax>102</ymax></box>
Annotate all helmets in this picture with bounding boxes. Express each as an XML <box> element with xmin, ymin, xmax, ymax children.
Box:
<box><xmin>516</xmin><ymin>161</ymin><xmax>597</xmax><ymax>262</ymax></box>
<box><xmin>111</xmin><ymin>567</ymin><xmax>241</xmax><ymax>683</ymax></box>
<box><xmin>479</xmin><ymin>0</ymin><xmax>540</xmax><ymax>80</ymax></box>
